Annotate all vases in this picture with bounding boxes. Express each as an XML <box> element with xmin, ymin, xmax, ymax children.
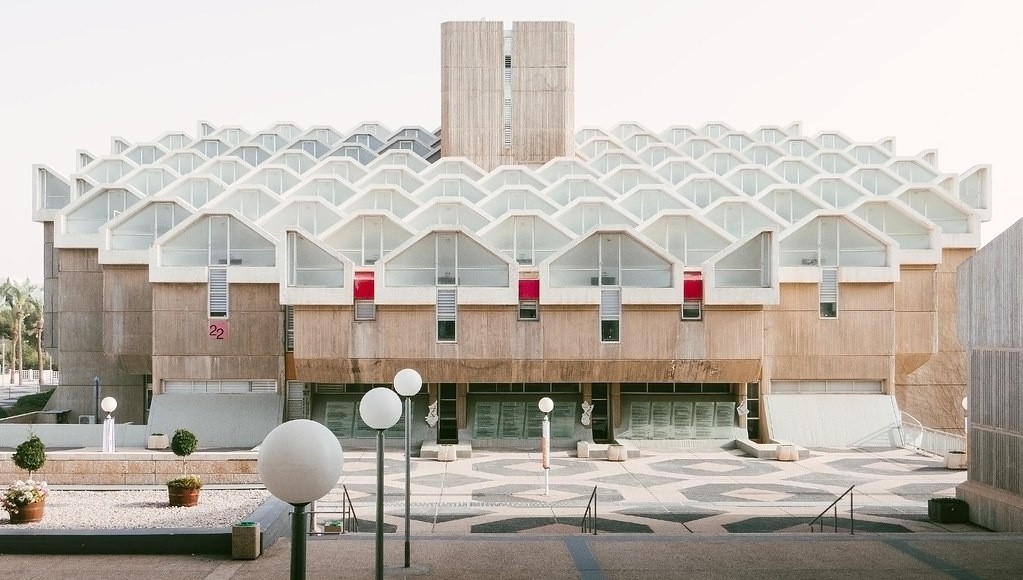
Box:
<box><xmin>231</xmin><ymin>522</ymin><xmax>260</xmax><ymax>560</ymax></box>
<box><xmin>8</xmin><ymin>501</ymin><xmax>44</xmax><ymax>522</ymax></box>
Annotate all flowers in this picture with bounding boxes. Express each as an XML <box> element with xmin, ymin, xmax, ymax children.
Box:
<box><xmin>0</xmin><ymin>479</ymin><xmax>48</xmax><ymax>514</ymax></box>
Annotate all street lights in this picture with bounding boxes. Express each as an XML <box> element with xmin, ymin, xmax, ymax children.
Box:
<box><xmin>538</xmin><ymin>397</ymin><xmax>554</xmax><ymax>495</ymax></box>
<box><xmin>101</xmin><ymin>396</ymin><xmax>117</xmax><ymax>452</ymax></box>
<box><xmin>358</xmin><ymin>387</ymin><xmax>402</xmax><ymax>580</ymax></box>
<box><xmin>393</xmin><ymin>368</ymin><xmax>422</xmax><ymax>568</ymax></box>
<box><xmin>259</xmin><ymin>418</ymin><xmax>343</xmax><ymax>580</ymax></box>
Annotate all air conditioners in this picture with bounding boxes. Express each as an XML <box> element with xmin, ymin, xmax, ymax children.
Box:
<box><xmin>79</xmin><ymin>415</ymin><xmax>96</xmax><ymax>424</ymax></box>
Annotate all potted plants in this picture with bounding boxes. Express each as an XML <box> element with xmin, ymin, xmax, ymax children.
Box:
<box><xmin>167</xmin><ymin>478</ymin><xmax>201</xmax><ymax>506</ymax></box>
<box><xmin>148</xmin><ymin>432</ymin><xmax>169</xmax><ymax>449</ymax></box>
<box><xmin>946</xmin><ymin>451</ymin><xmax>967</xmax><ymax>468</ymax></box>
<box><xmin>437</xmin><ymin>444</ymin><xmax>456</xmax><ymax>461</ymax></box>
<box><xmin>608</xmin><ymin>440</ymin><xmax>627</xmax><ymax>461</ymax></box>
<box><xmin>324</xmin><ymin>521</ymin><xmax>343</xmax><ymax>532</ymax></box>
<box><xmin>929</xmin><ymin>498</ymin><xmax>969</xmax><ymax>523</ymax></box>
<box><xmin>776</xmin><ymin>445</ymin><xmax>799</xmax><ymax>461</ymax></box>
